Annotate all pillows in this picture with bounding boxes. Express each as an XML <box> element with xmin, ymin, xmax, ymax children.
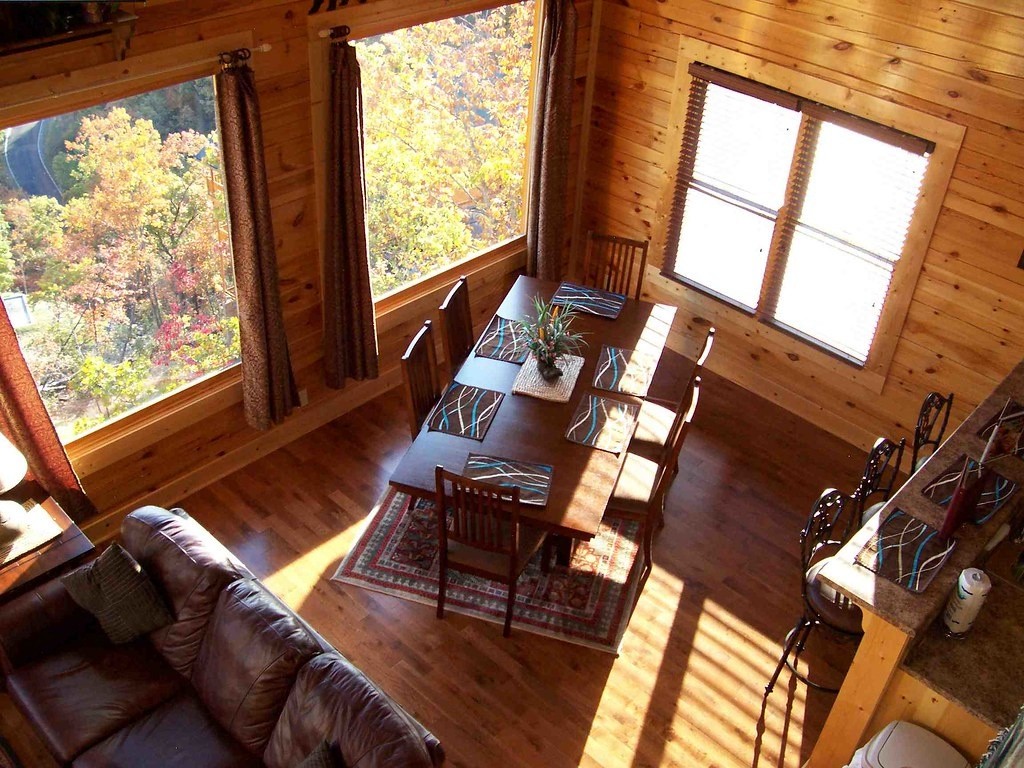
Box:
<box><xmin>60</xmin><ymin>540</ymin><xmax>175</xmax><ymax>643</ymax></box>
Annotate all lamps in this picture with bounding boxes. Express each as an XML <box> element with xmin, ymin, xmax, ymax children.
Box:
<box><xmin>0</xmin><ymin>429</ymin><xmax>28</xmax><ymax>544</ymax></box>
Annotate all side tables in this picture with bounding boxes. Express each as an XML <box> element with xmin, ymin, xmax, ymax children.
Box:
<box><xmin>0</xmin><ymin>478</ymin><xmax>100</xmax><ymax>599</ymax></box>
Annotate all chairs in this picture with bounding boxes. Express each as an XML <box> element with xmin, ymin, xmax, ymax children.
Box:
<box><xmin>402</xmin><ymin>319</ymin><xmax>441</xmax><ymax>443</ymax></box>
<box><xmin>855</xmin><ymin>435</ymin><xmax>906</xmax><ymax>526</ymax></box>
<box><xmin>582</xmin><ymin>230</ymin><xmax>649</xmax><ymax>301</ymax></box>
<box><xmin>628</xmin><ymin>327</ymin><xmax>716</xmax><ymax>459</ymax></box>
<box><xmin>603</xmin><ymin>375</ymin><xmax>701</xmax><ymax>569</ymax></box>
<box><xmin>435</xmin><ymin>463</ymin><xmax>554</xmax><ymax>636</ymax></box>
<box><xmin>764</xmin><ymin>487</ymin><xmax>866</xmax><ymax>695</ymax></box>
<box><xmin>439</xmin><ymin>276</ymin><xmax>474</xmax><ymax>379</ymax></box>
<box><xmin>910</xmin><ymin>390</ymin><xmax>954</xmax><ymax>473</ymax></box>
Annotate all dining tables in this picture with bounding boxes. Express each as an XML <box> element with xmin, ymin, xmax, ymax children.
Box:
<box><xmin>388</xmin><ymin>274</ymin><xmax>677</xmax><ymax>563</ymax></box>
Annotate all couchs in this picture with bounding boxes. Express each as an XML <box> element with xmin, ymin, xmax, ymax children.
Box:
<box><xmin>0</xmin><ymin>505</ymin><xmax>443</xmax><ymax>766</ymax></box>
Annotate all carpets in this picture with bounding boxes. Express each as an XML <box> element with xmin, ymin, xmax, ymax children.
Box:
<box><xmin>329</xmin><ymin>394</ymin><xmax>679</xmax><ymax>651</ymax></box>
